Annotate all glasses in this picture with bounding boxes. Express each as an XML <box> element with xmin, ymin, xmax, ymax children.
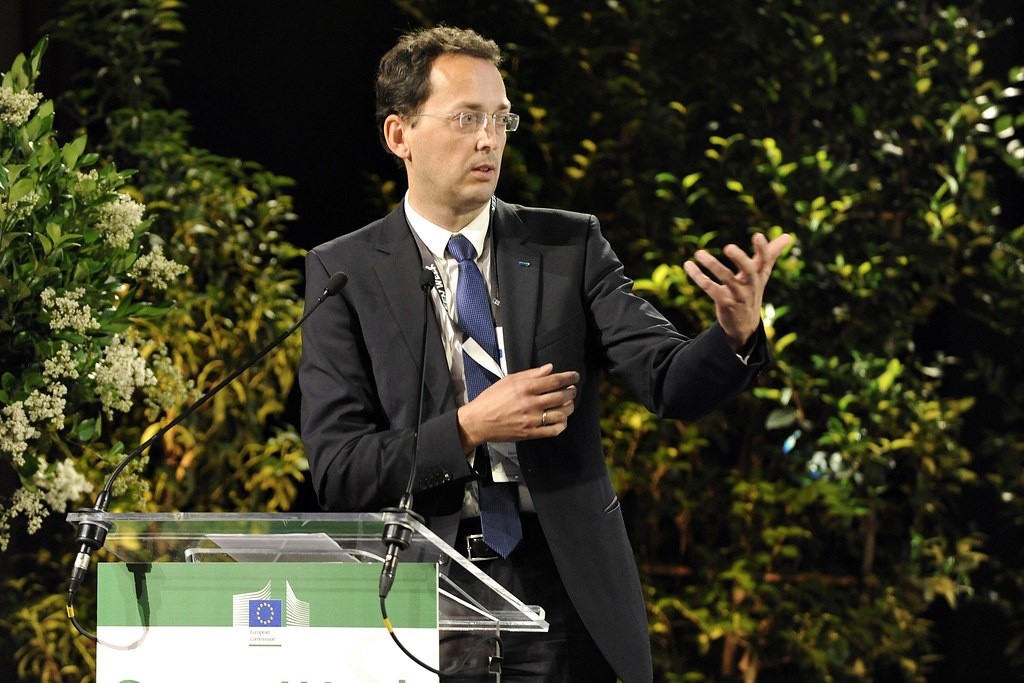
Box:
<box><xmin>404</xmin><ymin>109</ymin><xmax>520</xmax><ymax>133</ymax></box>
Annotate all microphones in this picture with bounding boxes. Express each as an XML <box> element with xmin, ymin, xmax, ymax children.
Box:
<box><xmin>381</xmin><ymin>270</ymin><xmax>435</xmax><ymax>523</ymax></box>
<box><xmin>76</xmin><ymin>271</ymin><xmax>348</xmax><ymax>515</ymax></box>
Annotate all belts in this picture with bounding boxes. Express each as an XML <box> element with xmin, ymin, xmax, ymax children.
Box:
<box><xmin>454</xmin><ymin>529</ymin><xmax>541</xmax><ymax>559</ymax></box>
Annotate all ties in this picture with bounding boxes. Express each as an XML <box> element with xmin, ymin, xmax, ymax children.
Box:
<box><xmin>446</xmin><ymin>234</ymin><xmax>523</xmax><ymax>559</ymax></box>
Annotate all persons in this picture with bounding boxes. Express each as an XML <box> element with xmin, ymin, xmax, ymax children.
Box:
<box><xmin>299</xmin><ymin>22</ymin><xmax>792</xmax><ymax>683</ymax></box>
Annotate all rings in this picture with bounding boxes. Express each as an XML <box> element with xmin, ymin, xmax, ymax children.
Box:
<box><xmin>542</xmin><ymin>412</ymin><xmax>547</xmax><ymax>425</ymax></box>
